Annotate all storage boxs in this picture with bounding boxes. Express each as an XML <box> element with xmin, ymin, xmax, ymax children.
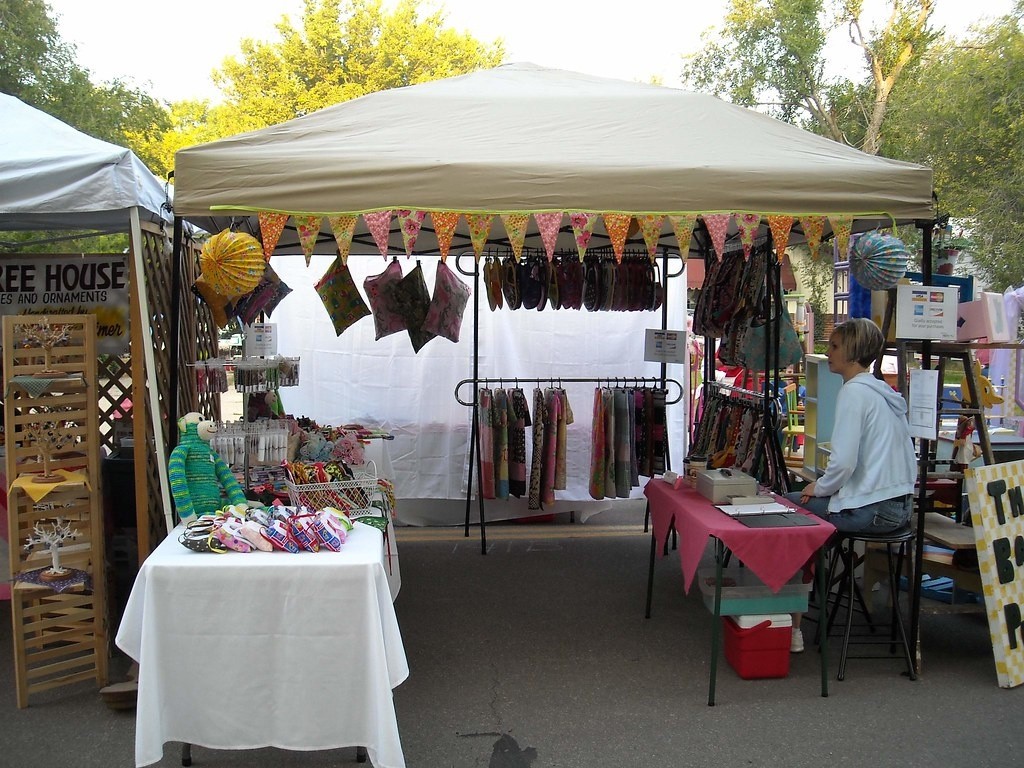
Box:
<box><xmin>119</xmin><ymin>436</ymin><xmax>135</xmax><ymax>458</ymax></box>
<box><xmin>721</xmin><ymin>613</ymin><xmax>793</xmax><ymax>680</ymax></box>
<box><xmin>698</xmin><ymin>567</ymin><xmax>813</xmax><ymax>614</ymax></box>
<box><xmin>696</xmin><ymin>469</ymin><xmax>756</xmax><ymax>503</ymax></box>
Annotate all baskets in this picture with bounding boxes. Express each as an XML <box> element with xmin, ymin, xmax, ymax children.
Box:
<box><xmin>284</xmin><ymin>460</ymin><xmax>378</xmax><ymax>515</ymax></box>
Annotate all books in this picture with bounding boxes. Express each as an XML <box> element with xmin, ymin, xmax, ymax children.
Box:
<box><xmin>712</xmin><ymin>501</ymin><xmax>821</xmax><ymax>528</ymax></box>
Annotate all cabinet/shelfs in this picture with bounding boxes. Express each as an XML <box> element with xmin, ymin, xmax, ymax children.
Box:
<box><xmin>1</xmin><ymin>313</ymin><xmax>111</xmax><ymax>706</ymax></box>
<box><xmin>802</xmin><ymin>354</ymin><xmax>844</xmax><ymax>479</ymax></box>
<box><xmin>187</xmin><ymin>357</ymin><xmax>300</xmax><ymax>498</ymax></box>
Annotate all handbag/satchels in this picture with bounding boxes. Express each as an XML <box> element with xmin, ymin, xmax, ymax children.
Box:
<box><xmin>177</xmin><ymin>503</ymin><xmax>353</xmax><ymax>554</ymax></box>
<box><xmin>701</xmin><ymin>250</ymin><xmax>804</xmax><ymax>373</ymax></box>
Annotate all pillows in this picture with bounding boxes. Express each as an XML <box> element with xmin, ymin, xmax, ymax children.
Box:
<box><xmin>192</xmin><ymin>245</ymin><xmax>470</xmax><ymax>354</ymax></box>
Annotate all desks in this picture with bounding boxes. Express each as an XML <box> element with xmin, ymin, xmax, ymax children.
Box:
<box><xmin>643</xmin><ymin>471</ymin><xmax>839</xmax><ymax>706</ymax></box>
<box><xmin>115</xmin><ymin>433</ymin><xmax>409</xmax><ymax>768</ymax></box>
<box><xmin>935</xmin><ymin>434</ymin><xmax>1024</xmax><ymax>492</ymax></box>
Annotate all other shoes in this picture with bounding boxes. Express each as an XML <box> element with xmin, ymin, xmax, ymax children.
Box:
<box><xmin>791</xmin><ymin>631</ymin><xmax>804</xmax><ymax>652</ymax></box>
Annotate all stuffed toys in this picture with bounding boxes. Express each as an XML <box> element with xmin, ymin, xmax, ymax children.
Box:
<box><xmin>168</xmin><ymin>411</ymin><xmax>246</xmax><ymax>530</ymax></box>
<box><xmin>248</xmin><ymin>391</ymin><xmax>280</xmax><ymax>423</ymax></box>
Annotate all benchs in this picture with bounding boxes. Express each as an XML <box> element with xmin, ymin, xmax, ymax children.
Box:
<box><xmin>864</xmin><ymin>539</ymin><xmax>982</xmax><ymax>614</ymax></box>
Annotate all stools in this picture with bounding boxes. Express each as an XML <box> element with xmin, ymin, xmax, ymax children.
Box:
<box><xmin>813</xmin><ymin>526</ymin><xmax>918</xmax><ymax>681</ymax></box>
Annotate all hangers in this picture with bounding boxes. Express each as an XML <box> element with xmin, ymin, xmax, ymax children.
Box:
<box><xmin>483</xmin><ymin>248</ymin><xmax>659</xmax><ymax>265</ymax></box>
<box><xmin>708</xmin><ymin>380</ymin><xmax>774</xmax><ymax>420</ymax></box>
<box><xmin>710</xmin><ymin>235</ymin><xmax>775</xmax><ymax>265</ymax></box>
<box><xmin>482</xmin><ymin>377</ymin><xmax>669</xmax><ymax>395</ymax></box>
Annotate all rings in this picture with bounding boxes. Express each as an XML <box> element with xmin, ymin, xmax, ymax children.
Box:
<box><xmin>802</xmin><ymin>489</ymin><xmax>804</xmax><ymax>491</ymax></box>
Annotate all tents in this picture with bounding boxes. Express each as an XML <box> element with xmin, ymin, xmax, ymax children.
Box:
<box><xmin>167</xmin><ymin>63</ymin><xmax>939</xmax><ymax>682</ymax></box>
<box><xmin>0</xmin><ymin>90</ymin><xmax>215</xmax><ymax>538</ymax></box>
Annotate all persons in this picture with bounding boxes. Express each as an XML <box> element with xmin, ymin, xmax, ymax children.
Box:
<box><xmin>781</xmin><ymin>318</ymin><xmax>918</xmax><ymax>652</ymax></box>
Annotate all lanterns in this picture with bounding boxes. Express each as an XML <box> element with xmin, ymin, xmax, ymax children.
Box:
<box><xmin>847</xmin><ymin>227</ymin><xmax>909</xmax><ymax>292</ymax></box>
<box><xmin>199</xmin><ymin>226</ymin><xmax>266</xmax><ymax>297</ymax></box>
